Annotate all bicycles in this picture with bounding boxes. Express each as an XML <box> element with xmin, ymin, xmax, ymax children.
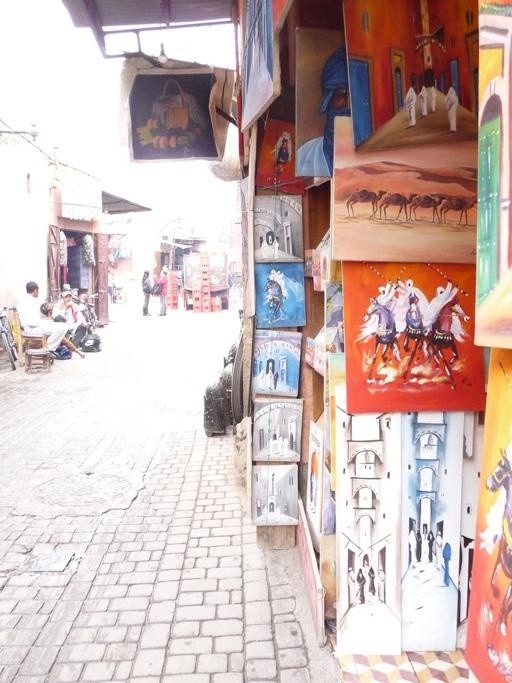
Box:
<box><xmin>108</xmin><ymin>276</ymin><xmax>126</xmax><ymax>304</ymax></box>
<box><xmin>0</xmin><ymin>305</ymin><xmax>19</xmax><ymax>370</ymax></box>
<box><xmin>69</xmin><ymin>293</ymin><xmax>101</xmax><ymax>331</ymax></box>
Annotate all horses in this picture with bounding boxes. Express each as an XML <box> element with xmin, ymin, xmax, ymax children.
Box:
<box><xmin>403</xmin><ymin>291</ymin><xmax>425</xmax><ymax>384</ymax></box>
<box><xmin>426</xmin><ymin>294</ymin><xmax>471</xmax><ymax>390</ymax></box>
<box><xmin>484</xmin><ymin>447</ymin><xmax>512</xmax><ymax>637</ymax></box>
<box><xmin>362</xmin><ymin>297</ymin><xmax>396</xmax><ymax>380</ymax></box>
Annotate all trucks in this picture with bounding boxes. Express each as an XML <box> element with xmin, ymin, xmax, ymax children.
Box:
<box><xmin>181</xmin><ymin>249</ymin><xmax>229</xmax><ymax>311</ymax></box>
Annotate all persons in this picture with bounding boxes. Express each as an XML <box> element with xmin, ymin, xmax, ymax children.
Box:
<box><xmin>153</xmin><ymin>272</ymin><xmax>168</xmax><ymax>316</ymax></box>
<box><xmin>17</xmin><ymin>281</ymin><xmax>68</xmax><ymax>358</ymax></box>
<box><xmin>142</xmin><ymin>271</ymin><xmax>152</xmax><ymax>316</ymax></box>
<box><xmin>51</xmin><ymin>291</ymin><xmax>87</xmax><ymax>348</ymax></box>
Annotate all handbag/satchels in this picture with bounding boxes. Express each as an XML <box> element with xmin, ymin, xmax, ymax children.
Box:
<box><xmin>81</xmin><ymin>334</ymin><xmax>102</xmax><ymax>352</ymax></box>
<box><xmin>151</xmin><ymin>283</ymin><xmax>162</xmax><ymax>295</ymax></box>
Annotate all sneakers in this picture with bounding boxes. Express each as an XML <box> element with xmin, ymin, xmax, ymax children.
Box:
<box><xmin>47</xmin><ymin>349</ymin><xmax>59</xmax><ymax>359</ymax></box>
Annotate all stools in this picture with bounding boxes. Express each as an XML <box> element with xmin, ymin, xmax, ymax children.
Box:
<box><xmin>26</xmin><ymin>348</ymin><xmax>51</xmax><ymax>373</ymax></box>
<box><xmin>21</xmin><ymin>332</ymin><xmax>46</xmax><ymax>348</ymax></box>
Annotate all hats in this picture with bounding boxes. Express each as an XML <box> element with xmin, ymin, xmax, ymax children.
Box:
<box><xmin>62</xmin><ymin>291</ymin><xmax>72</xmax><ymax>298</ymax></box>
